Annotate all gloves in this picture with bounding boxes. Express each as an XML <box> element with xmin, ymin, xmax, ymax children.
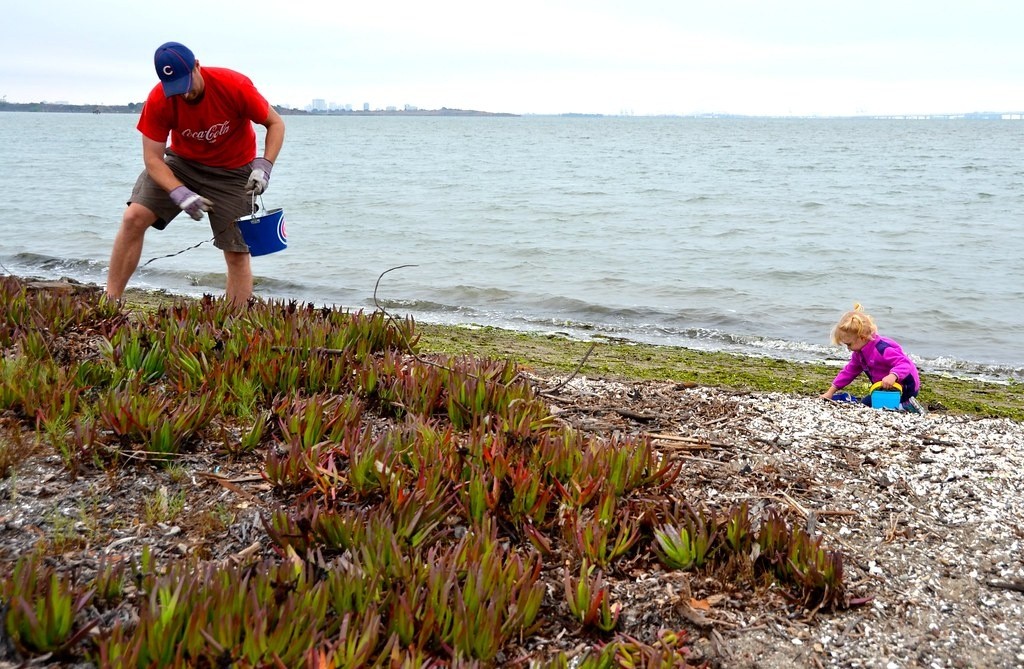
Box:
<box><xmin>244</xmin><ymin>156</ymin><xmax>273</xmax><ymax>196</ymax></box>
<box><xmin>169</xmin><ymin>184</ymin><xmax>215</xmax><ymax>221</ymax></box>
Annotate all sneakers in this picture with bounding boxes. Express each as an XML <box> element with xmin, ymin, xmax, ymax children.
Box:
<box><xmin>902</xmin><ymin>396</ymin><xmax>928</xmax><ymax>416</ymax></box>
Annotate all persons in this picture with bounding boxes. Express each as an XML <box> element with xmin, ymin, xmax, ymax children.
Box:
<box><xmin>817</xmin><ymin>303</ymin><xmax>927</xmax><ymax>415</ymax></box>
<box><xmin>106</xmin><ymin>43</ymin><xmax>285</xmax><ymax>315</ymax></box>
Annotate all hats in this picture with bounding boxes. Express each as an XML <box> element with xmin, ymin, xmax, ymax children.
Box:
<box><xmin>154</xmin><ymin>42</ymin><xmax>195</xmax><ymax>99</ymax></box>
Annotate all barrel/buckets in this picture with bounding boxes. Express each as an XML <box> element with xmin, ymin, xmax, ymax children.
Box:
<box><xmin>233</xmin><ymin>190</ymin><xmax>287</xmax><ymax>257</ymax></box>
<box><xmin>870</xmin><ymin>381</ymin><xmax>902</xmax><ymax>410</ymax></box>
